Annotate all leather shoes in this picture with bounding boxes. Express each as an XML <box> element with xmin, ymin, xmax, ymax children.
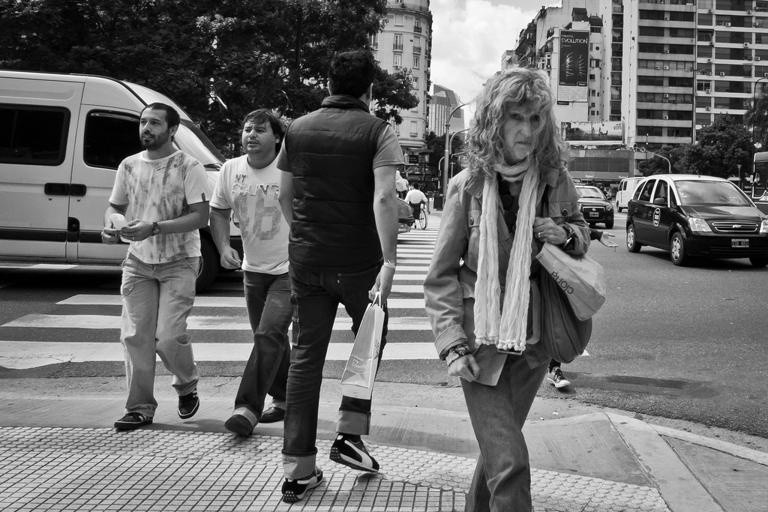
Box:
<box><xmin>260</xmin><ymin>406</ymin><xmax>287</xmax><ymax>424</ymax></box>
<box><xmin>223</xmin><ymin>414</ymin><xmax>256</xmax><ymax>438</ymax></box>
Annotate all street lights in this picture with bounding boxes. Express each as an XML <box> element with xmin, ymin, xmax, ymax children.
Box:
<box><xmin>443</xmin><ymin>100</ymin><xmax>473</xmax><ymax>212</ymax></box>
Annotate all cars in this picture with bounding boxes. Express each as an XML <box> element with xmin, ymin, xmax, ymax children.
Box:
<box><xmin>576</xmin><ymin>186</ymin><xmax>613</xmax><ymax>228</ymax></box>
<box><xmin>627</xmin><ymin>174</ymin><xmax>768</xmax><ymax>268</ymax></box>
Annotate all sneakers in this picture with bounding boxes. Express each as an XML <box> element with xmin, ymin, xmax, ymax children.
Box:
<box><xmin>546</xmin><ymin>365</ymin><xmax>573</xmax><ymax>390</ymax></box>
<box><xmin>176</xmin><ymin>386</ymin><xmax>201</xmax><ymax>418</ymax></box>
<box><xmin>277</xmin><ymin>468</ymin><xmax>328</xmax><ymax>506</ymax></box>
<box><xmin>326</xmin><ymin>433</ymin><xmax>383</xmax><ymax>480</ymax></box>
<box><xmin>113</xmin><ymin>412</ymin><xmax>155</xmax><ymax>432</ymax></box>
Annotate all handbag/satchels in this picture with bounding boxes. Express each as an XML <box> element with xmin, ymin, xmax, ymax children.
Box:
<box><xmin>338</xmin><ymin>290</ymin><xmax>387</xmax><ymax>404</ymax></box>
<box><xmin>535</xmin><ymin>242</ymin><xmax>606</xmax><ymax>322</ymax></box>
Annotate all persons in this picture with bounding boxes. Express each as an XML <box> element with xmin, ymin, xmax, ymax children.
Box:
<box><xmin>101</xmin><ymin>103</ymin><xmax>210</xmax><ymax>430</ymax></box>
<box><xmin>423</xmin><ymin>67</ymin><xmax>607</xmax><ymax>512</ymax></box>
<box><xmin>212</xmin><ymin>108</ymin><xmax>296</xmax><ymax>437</ymax></box>
<box><xmin>277</xmin><ymin>49</ymin><xmax>404</xmax><ymax>503</ymax></box>
<box><xmin>404</xmin><ymin>183</ymin><xmax>430</xmax><ymax>229</ymax></box>
<box><xmin>548</xmin><ymin>224</ymin><xmax>618</xmax><ymax>388</ymax></box>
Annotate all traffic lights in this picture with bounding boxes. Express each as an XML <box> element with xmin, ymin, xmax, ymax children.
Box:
<box><xmin>632</xmin><ymin>144</ymin><xmax>637</xmax><ymax>152</ymax></box>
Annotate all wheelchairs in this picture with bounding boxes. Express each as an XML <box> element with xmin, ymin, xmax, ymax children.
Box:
<box><xmin>418</xmin><ymin>199</ymin><xmax>427</xmax><ymax>230</ymax></box>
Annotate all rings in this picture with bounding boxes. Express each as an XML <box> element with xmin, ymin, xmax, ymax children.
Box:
<box><xmin>131</xmin><ymin>236</ymin><xmax>137</xmax><ymax>241</ymax></box>
<box><xmin>537</xmin><ymin>231</ymin><xmax>541</xmax><ymax>238</ymax></box>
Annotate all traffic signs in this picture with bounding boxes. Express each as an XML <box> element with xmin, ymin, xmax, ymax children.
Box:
<box><xmin>640</xmin><ymin>147</ymin><xmax>645</xmax><ymax>152</ymax></box>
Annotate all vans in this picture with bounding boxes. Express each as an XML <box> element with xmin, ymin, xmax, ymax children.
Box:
<box><xmin>616</xmin><ymin>178</ymin><xmax>644</xmax><ymax>212</ymax></box>
<box><xmin>0</xmin><ymin>71</ymin><xmax>239</xmax><ymax>295</ymax></box>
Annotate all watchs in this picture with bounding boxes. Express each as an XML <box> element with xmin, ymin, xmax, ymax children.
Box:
<box><xmin>153</xmin><ymin>216</ymin><xmax>162</xmax><ymax>235</ymax></box>
